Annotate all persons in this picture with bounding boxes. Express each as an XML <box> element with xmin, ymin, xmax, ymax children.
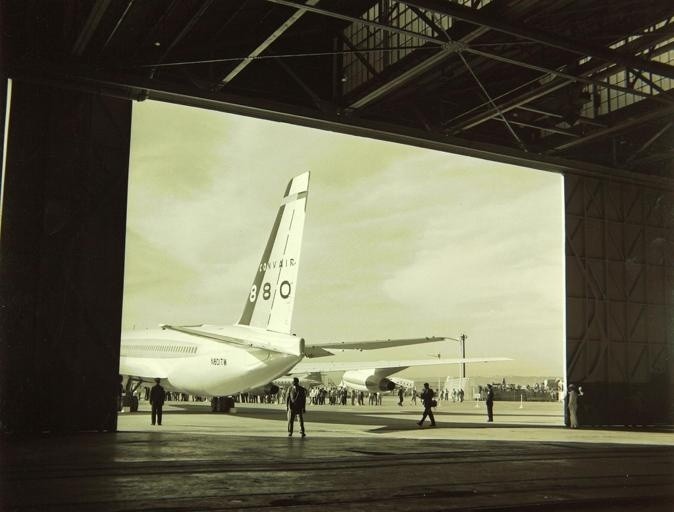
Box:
<box><xmin>484</xmin><ymin>383</ymin><xmax>493</xmax><ymax>422</ymax></box>
<box><xmin>567</xmin><ymin>383</ymin><xmax>582</xmax><ymax>428</ymax></box>
<box><xmin>412</xmin><ymin>387</ymin><xmax>416</xmax><ymax>401</ymax></box>
<box><xmin>286</xmin><ymin>377</ymin><xmax>305</xmax><ymax>436</ymax></box>
<box><xmin>308</xmin><ymin>386</ymin><xmax>382</xmax><ymax>406</ymax></box>
<box><xmin>440</xmin><ymin>388</ymin><xmax>464</xmax><ymax>402</ymax></box>
<box><xmin>138</xmin><ymin>386</ymin><xmax>148</xmax><ymax>400</ymax></box>
<box><xmin>416</xmin><ymin>382</ymin><xmax>436</xmax><ymax>426</ymax></box>
<box><xmin>167</xmin><ymin>391</ymin><xmax>207</xmax><ymax>402</ymax></box>
<box><xmin>232</xmin><ymin>388</ymin><xmax>283</xmax><ymax>404</ymax></box>
<box><xmin>149</xmin><ymin>378</ymin><xmax>165</xmax><ymax>425</ymax></box>
<box><xmin>398</xmin><ymin>386</ymin><xmax>403</xmax><ymax>406</ymax></box>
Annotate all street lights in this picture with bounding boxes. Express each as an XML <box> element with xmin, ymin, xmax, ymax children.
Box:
<box><xmin>459</xmin><ymin>334</ymin><xmax>468</xmax><ymax>377</ymax></box>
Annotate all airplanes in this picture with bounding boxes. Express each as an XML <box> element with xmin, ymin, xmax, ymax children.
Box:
<box><xmin>271</xmin><ymin>371</ymin><xmax>328</xmax><ymax>397</ymax></box>
<box><xmin>117</xmin><ymin>170</ymin><xmax>515</xmax><ymax>413</ymax></box>
